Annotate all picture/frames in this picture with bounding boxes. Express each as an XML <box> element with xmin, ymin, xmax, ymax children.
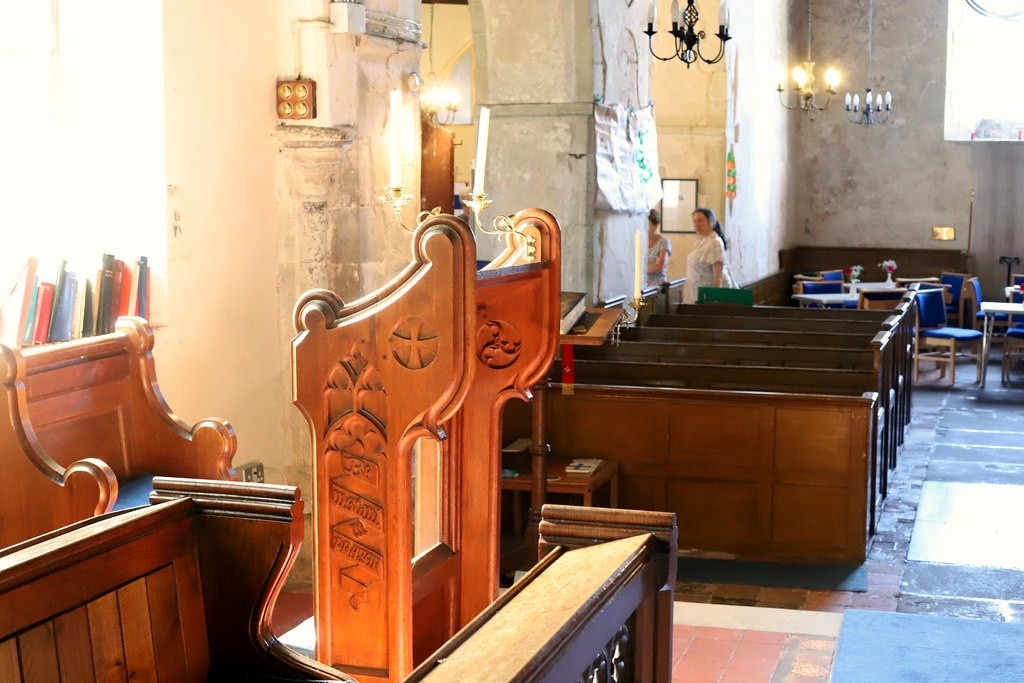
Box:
<box><xmin>661</xmin><ymin>177</ymin><xmax>697</xmax><ymax>234</ymax></box>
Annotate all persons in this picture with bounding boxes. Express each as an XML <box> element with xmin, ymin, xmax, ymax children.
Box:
<box><xmin>647</xmin><ymin>208</ymin><xmax>673</xmax><ymax>287</ymax></box>
<box><xmin>686</xmin><ymin>208</ymin><xmax>738</xmax><ymax>304</ymax></box>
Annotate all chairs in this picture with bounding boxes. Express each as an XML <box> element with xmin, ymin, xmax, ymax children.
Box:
<box><xmin>793</xmin><ymin>269</ymin><xmax>859</xmax><ymax>309</ymax></box>
<box><xmin>894</xmin><ymin>274</ymin><xmax>1024</xmax><ymax>389</ymax></box>
<box><xmin>0</xmin><ymin>317</ymin><xmax>239</xmax><ymax>553</ymax></box>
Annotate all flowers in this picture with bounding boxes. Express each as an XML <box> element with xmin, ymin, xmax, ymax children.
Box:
<box><xmin>878</xmin><ymin>260</ymin><xmax>898</xmax><ymax>271</ymax></box>
<box><xmin>844</xmin><ymin>264</ymin><xmax>863</xmax><ymax>282</ymax></box>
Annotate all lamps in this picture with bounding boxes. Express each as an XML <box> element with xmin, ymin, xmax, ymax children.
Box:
<box><xmin>842</xmin><ymin>0</ymin><xmax>894</xmax><ymax>127</ymax></box>
<box><xmin>642</xmin><ymin>0</ymin><xmax>731</xmax><ymax>67</ymax></box>
<box><xmin>775</xmin><ymin>0</ymin><xmax>841</xmax><ymax>112</ymax></box>
<box><xmin>414</xmin><ymin>1</ymin><xmax>459</xmax><ymax>128</ymax></box>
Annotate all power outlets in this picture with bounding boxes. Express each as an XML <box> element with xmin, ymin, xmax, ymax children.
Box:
<box><xmin>242</xmin><ymin>463</ymin><xmax>265</xmax><ymax>484</ymax></box>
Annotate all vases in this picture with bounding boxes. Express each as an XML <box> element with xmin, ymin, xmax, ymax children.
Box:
<box><xmin>849</xmin><ymin>284</ymin><xmax>859</xmax><ymax>301</ymax></box>
<box><xmin>884</xmin><ymin>273</ymin><xmax>895</xmax><ymax>289</ymax></box>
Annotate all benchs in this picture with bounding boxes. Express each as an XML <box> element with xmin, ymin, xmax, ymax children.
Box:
<box><xmin>0</xmin><ymin>476</ymin><xmax>356</xmax><ymax>683</ymax></box>
<box><xmin>502</xmin><ymin>292</ymin><xmax>918</xmax><ymax>567</ymax></box>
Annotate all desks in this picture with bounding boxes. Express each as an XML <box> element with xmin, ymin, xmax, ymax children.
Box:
<box><xmin>979</xmin><ymin>302</ymin><xmax>1024</xmax><ymax>390</ymax></box>
<box><xmin>499</xmin><ymin>462</ymin><xmax>618</xmax><ymax>538</ymax></box>
<box><xmin>841</xmin><ymin>281</ymin><xmax>901</xmax><ymax>295</ymax></box>
<box><xmin>792</xmin><ymin>295</ymin><xmax>860</xmax><ymax>309</ymax></box>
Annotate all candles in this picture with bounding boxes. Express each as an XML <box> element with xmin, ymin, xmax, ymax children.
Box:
<box><xmin>474</xmin><ymin>103</ymin><xmax>491</xmax><ymax>198</ymax></box>
<box><xmin>633</xmin><ymin>230</ymin><xmax>643</xmax><ymax>303</ymax></box>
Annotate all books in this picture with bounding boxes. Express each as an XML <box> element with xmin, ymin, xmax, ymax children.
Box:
<box><xmin>0</xmin><ymin>251</ymin><xmax>150</xmax><ymax>348</ymax></box>
